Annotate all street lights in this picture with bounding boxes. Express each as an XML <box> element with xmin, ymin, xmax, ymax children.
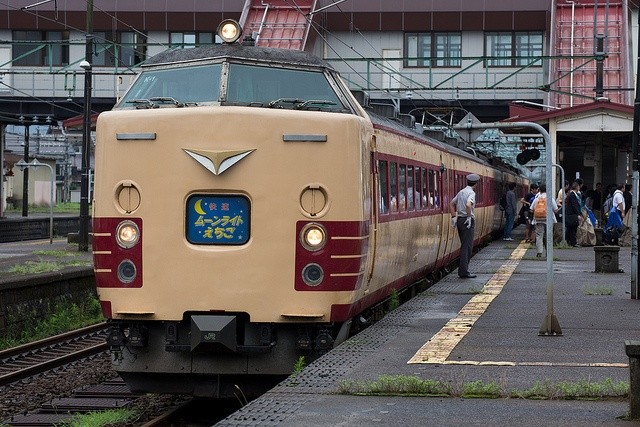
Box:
<box><xmin>79</xmin><ymin>60</ymin><xmax>90</xmax><ymax>250</ymax></box>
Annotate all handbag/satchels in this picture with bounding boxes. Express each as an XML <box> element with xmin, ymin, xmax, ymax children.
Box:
<box><xmin>565</xmin><ymin>214</ymin><xmax>579</xmax><ymax>227</ymax></box>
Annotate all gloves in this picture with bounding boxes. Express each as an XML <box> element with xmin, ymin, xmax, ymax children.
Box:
<box><xmin>451</xmin><ymin>215</ymin><xmax>458</xmax><ymax>232</ymax></box>
<box><xmin>463</xmin><ymin>217</ymin><xmax>472</xmax><ymax>229</ymax></box>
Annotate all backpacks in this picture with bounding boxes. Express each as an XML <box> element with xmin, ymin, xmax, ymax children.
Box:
<box><xmin>518</xmin><ymin>202</ymin><xmax>527</xmax><ymax>225</ymax></box>
<box><xmin>604</xmin><ymin>192</ymin><xmax>623</xmax><ymax>220</ymax></box>
<box><xmin>499</xmin><ymin>194</ymin><xmax>506</xmax><ymax>211</ymax></box>
<box><xmin>534</xmin><ymin>197</ymin><xmax>547</xmax><ymax>222</ymax></box>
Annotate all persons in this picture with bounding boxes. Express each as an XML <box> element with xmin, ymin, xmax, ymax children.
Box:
<box><xmin>520</xmin><ymin>183</ymin><xmax>534</xmax><ymax>243</ymax></box>
<box><xmin>450</xmin><ymin>174</ymin><xmax>480</xmax><ymax>278</ymax></box>
<box><xmin>530</xmin><ymin>185</ymin><xmax>558</xmax><ymax>257</ymax></box>
<box><xmin>503</xmin><ymin>183</ymin><xmax>517</xmax><ymax>241</ymax></box>
<box><xmin>559</xmin><ymin>180</ymin><xmax>633</xmax><ymax>242</ymax></box>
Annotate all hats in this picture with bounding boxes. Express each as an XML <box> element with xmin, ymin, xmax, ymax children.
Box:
<box><xmin>466</xmin><ymin>173</ymin><xmax>481</xmax><ymax>184</ymax></box>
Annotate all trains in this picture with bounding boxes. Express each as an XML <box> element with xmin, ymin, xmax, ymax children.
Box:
<box><xmin>90</xmin><ymin>20</ymin><xmax>534</xmax><ymax>397</ymax></box>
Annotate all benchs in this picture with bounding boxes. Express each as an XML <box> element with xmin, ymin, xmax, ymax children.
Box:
<box><xmin>594</xmin><ymin>229</ymin><xmax>624</xmax><ymax>273</ymax></box>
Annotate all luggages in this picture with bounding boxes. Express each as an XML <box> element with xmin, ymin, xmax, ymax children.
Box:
<box><xmin>604</xmin><ymin>212</ymin><xmax>619</xmax><ymax>245</ymax></box>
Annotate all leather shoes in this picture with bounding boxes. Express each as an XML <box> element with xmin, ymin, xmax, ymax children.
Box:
<box><xmin>460</xmin><ymin>274</ymin><xmax>477</xmax><ymax>278</ymax></box>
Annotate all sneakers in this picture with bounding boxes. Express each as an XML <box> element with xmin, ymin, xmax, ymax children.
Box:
<box><xmin>536</xmin><ymin>253</ymin><xmax>541</xmax><ymax>257</ymax></box>
<box><xmin>505</xmin><ymin>237</ymin><xmax>514</xmax><ymax>241</ymax></box>
<box><xmin>503</xmin><ymin>237</ymin><xmax>506</xmax><ymax>240</ymax></box>
